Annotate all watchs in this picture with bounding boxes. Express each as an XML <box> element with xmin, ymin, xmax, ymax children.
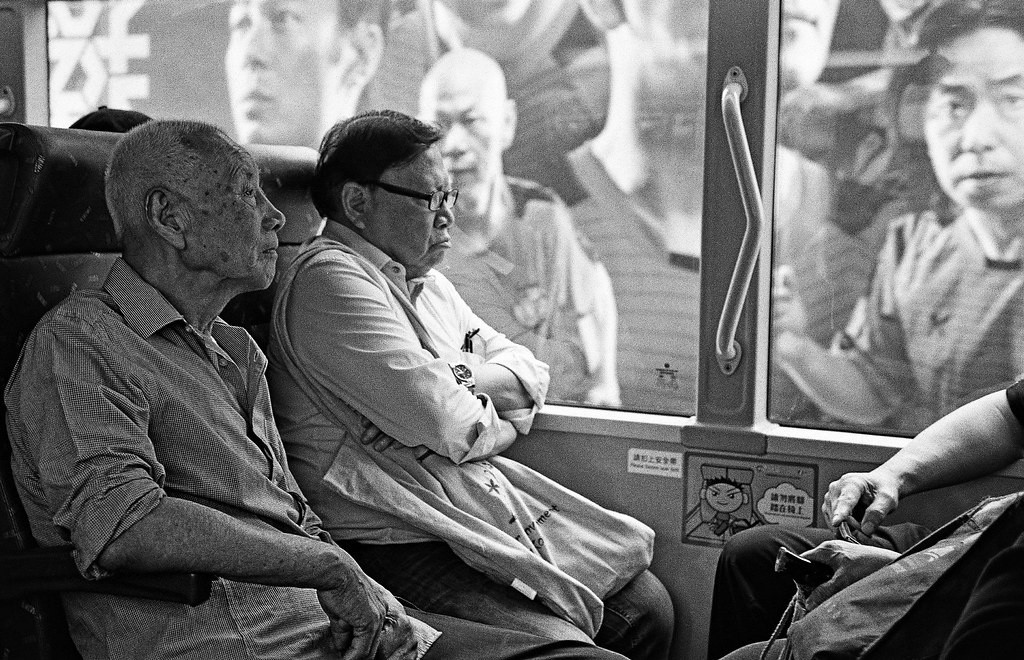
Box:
<box><xmin>448</xmin><ymin>363</ymin><xmax>476</xmax><ymax>394</ymax></box>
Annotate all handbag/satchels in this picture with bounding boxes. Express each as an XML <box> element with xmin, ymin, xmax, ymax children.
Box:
<box><xmin>270</xmin><ymin>237</ymin><xmax>656</xmax><ymax>639</ymax></box>
<box><xmin>786</xmin><ymin>490</ymin><xmax>1022</xmax><ymax>659</ymax></box>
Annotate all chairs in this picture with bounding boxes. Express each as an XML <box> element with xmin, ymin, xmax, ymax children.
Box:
<box><xmin>219</xmin><ymin>143</ymin><xmax>330</xmax><ymax>342</ymax></box>
<box><xmin>0</xmin><ymin>123</ymin><xmax>214</xmax><ymax>658</ymax></box>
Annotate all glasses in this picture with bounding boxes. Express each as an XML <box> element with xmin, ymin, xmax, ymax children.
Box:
<box><xmin>378</xmin><ymin>180</ymin><xmax>459</xmax><ymax>211</ymax></box>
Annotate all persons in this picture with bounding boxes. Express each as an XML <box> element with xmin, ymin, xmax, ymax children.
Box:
<box><xmin>217</xmin><ymin>0</ymin><xmax>874</xmax><ymax>442</ymax></box>
<box><xmin>265</xmin><ymin>109</ymin><xmax>673</xmax><ymax>660</ymax></box>
<box><xmin>706</xmin><ymin>378</ymin><xmax>1024</xmax><ymax>660</ymax></box>
<box><xmin>0</xmin><ymin>120</ymin><xmax>630</xmax><ymax>660</ymax></box>
<box><xmin>769</xmin><ymin>0</ymin><xmax>1024</xmax><ymax>437</ymax></box>
